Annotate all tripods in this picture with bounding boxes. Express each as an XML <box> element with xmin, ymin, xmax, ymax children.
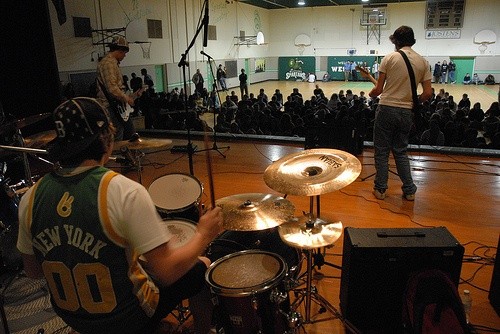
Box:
<box><xmin>194</xmin><ymin>58</ymin><xmax>230</xmax><ymax>158</ymax></box>
<box><xmin>291</xmin><ymin>192</ymin><xmax>342</xmax><ymax>323</ymax></box>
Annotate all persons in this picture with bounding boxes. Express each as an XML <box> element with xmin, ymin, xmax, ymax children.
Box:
<box><xmin>214</xmin><ymin>84</ymin><xmax>500</xmax><ymax>150</ymax></box>
<box><xmin>322</xmin><ymin>71</ymin><xmax>330</xmax><ymax>82</ymax></box>
<box><xmin>433</xmin><ymin>61</ymin><xmax>456</xmax><ymax>84</ymax></box>
<box><xmin>217</xmin><ymin>64</ymin><xmax>229</xmax><ymax>92</ymax></box>
<box><xmin>239</xmin><ymin>68</ymin><xmax>247</xmax><ymax>100</ymax></box>
<box><xmin>122</xmin><ymin>68</ymin><xmax>213</xmax><ymax>132</ymax></box>
<box><xmin>463</xmin><ymin>73</ymin><xmax>472</xmax><ymax>84</ymax></box>
<box><xmin>369</xmin><ymin>25</ymin><xmax>430</xmax><ymax>201</ymax></box>
<box><xmin>16</xmin><ymin>97</ymin><xmax>223</xmax><ymax>334</ymax></box>
<box><xmin>96</xmin><ymin>36</ymin><xmax>134</xmax><ymax>170</ymax></box>
<box><xmin>484</xmin><ymin>74</ymin><xmax>495</xmax><ymax>85</ymax></box>
<box><xmin>302</xmin><ymin>72</ymin><xmax>316</xmax><ymax>83</ymax></box>
<box><xmin>343</xmin><ymin>60</ymin><xmax>379</xmax><ymax>82</ymax></box>
<box><xmin>473</xmin><ymin>73</ymin><xmax>479</xmax><ymax>85</ymax></box>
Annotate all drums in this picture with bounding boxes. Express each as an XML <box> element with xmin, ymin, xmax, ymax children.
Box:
<box><xmin>206</xmin><ymin>226</ymin><xmax>302</xmax><ymax>285</ymax></box>
<box><xmin>138</xmin><ymin>218</ymin><xmax>198</xmax><ymax>277</ymax></box>
<box><xmin>204</xmin><ymin>249</ymin><xmax>293</xmax><ymax>334</ymax></box>
<box><xmin>148</xmin><ymin>172</ymin><xmax>203</xmax><ymax>228</ymax></box>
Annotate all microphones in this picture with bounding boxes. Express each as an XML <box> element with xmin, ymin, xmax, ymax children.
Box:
<box><xmin>203</xmin><ymin>0</ymin><xmax>209</xmax><ymax>47</ymax></box>
<box><xmin>200</xmin><ymin>51</ymin><xmax>212</xmax><ymax>58</ymax></box>
<box><xmin>120</xmin><ymin>145</ymin><xmax>137</xmax><ymax>167</ymax></box>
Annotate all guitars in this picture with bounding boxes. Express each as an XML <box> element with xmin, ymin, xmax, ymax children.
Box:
<box><xmin>112</xmin><ymin>85</ymin><xmax>148</xmax><ymax>123</ymax></box>
<box><xmin>354</xmin><ymin>65</ymin><xmax>377</xmax><ymax>85</ymax></box>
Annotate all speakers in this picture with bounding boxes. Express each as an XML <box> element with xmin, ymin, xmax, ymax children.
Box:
<box><xmin>339</xmin><ymin>225</ymin><xmax>465</xmax><ymax>328</ymax></box>
<box><xmin>305</xmin><ymin>126</ymin><xmax>364</xmax><ymax>154</ymax></box>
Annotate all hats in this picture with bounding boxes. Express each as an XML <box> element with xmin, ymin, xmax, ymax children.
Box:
<box><xmin>107</xmin><ymin>36</ymin><xmax>129</xmax><ymax>50</ymax></box>
<box><xmin>46</xmin><ymin>97</ymin><xmax>107</xmax><ymax>159</ymax></box>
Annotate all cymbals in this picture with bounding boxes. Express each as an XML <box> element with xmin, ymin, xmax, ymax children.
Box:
<box><xmin>110</xmin><ymin>138</ymin><xmax>173</xmax><ymax>150</ymax></box>
<box><xmin>278</xmin><ymin>213</ymin><xmax>343</xmax><ymax>251</ymax></box>
<box><xmin>0</xmin><ymin>112</ymin><xmax>50</xmax><ymax>133</ymax></box>
<box><xmin>207</xmin><ymin>192</ymin><xmax>296</xmax><ymax>232</ymax></box>
<box><xmin>263</xmin><ymin>148</ymin><xmax>362</xmax><ymax>197</ymax></box>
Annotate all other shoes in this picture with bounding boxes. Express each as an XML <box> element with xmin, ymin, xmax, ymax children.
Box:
<box><xmin>405</xmin><ymin>194</ymin><xmax>415</xmax><ymax>200</ymax></box>
<box><xmin>121</xmin><ymin>161</ymin><xmax>143</xmax><ymax>170</ymax></box>
<box><xmin>373</xmin><ymin>190</ymin><xmax>386</xmax><ymax>200</ymax></box>
